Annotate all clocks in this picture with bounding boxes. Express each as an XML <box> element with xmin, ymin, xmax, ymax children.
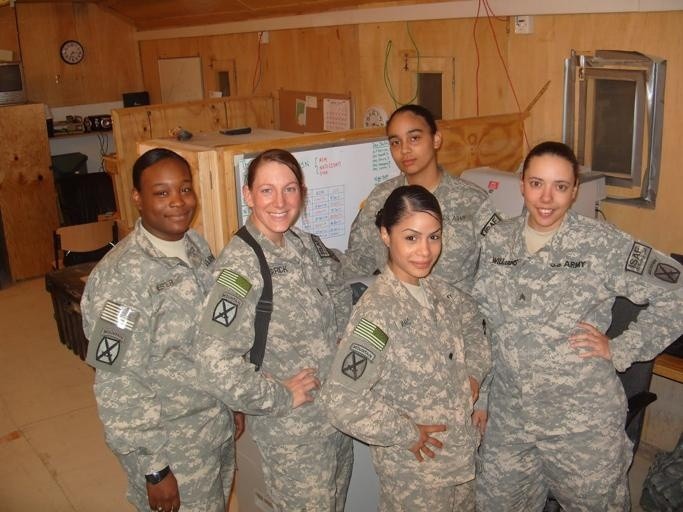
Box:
<box><xmin>61</xmin><ymin>39</ymin><xmax>85</xmax><ymax>66</ymax></box>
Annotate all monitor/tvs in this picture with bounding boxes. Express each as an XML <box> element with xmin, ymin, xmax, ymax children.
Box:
<box><xmin>0</xmin><ymin>63</ymin><xmax>28</xmax><ymax>105</ymax></box>
<box><xmin>121</xmin><ymin>92</ymin><xmax>150</xmax><ymax>108</ymax></box>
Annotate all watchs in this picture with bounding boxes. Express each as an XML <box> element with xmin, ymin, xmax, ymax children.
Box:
<box><xmin>144</xmin><ymin>465</ymin><xmax>172</xmax><ymax>483</ymax></box>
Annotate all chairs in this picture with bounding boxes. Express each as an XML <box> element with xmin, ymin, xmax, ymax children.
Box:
<box><xmin>545</xmin><ymin>294</ymin><xmax>662</xmax><ymax>508</ymax></box>
<box><xmin>50</xmin><ymin>219</ymin><xmax>119</xmax><ymax>272</ymax></box>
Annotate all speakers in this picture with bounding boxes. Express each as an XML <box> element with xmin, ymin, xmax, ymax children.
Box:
<box><xmin>83</xmin><ymin>114</ymin><xmax>113</xmax><ymax>132</ymax></box>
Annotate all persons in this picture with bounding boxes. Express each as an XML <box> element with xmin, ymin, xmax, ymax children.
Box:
<box><xmin>79</xmin><ymin>148</ymin><xmax>250</xmax><ymax>511</ymax></box>
<box><xmin>339</xmin><ymin>104</ymin><xmax>508</xmax><ymax>292</ymax></box>
<box><xmin>198</xmin><ymin>149</ymin><xmax>354</xmax><ymax>512</ymax></box>
<box><xmin>318</xmin><ymin>185</ymin><xmax>493</xmax><ymax>511</ymax></box>
<box><xmin>470</xmin><ymin>141</ymin><xmax>683</xmax><ymax>512</ymax></box>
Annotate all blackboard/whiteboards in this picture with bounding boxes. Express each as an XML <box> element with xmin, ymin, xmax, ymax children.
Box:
<box><xmin>233</xmin><ymin>136</ymin><xmax>407</xmax><ymax>255</ymax></box>
